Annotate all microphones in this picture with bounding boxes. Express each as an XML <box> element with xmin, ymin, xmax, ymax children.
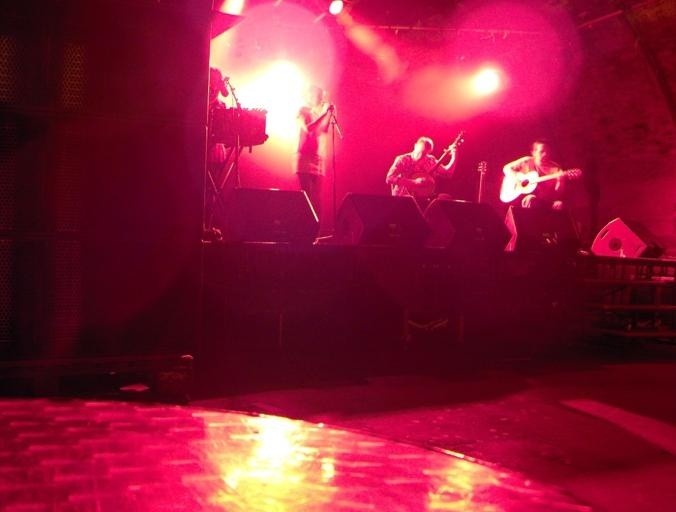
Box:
<box><xmin>328</xmin><ymin>104</ymin><xmax>335</xmax><ymax>111</ymax></box>
<box><xmin>223</xmin><ymin>76</ymin><xmax>230</xmax><ymax>82</ymax></box>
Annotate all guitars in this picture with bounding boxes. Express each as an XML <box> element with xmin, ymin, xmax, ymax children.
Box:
<box><xmin>500</xmin><ymin>169</ymin><xmax>582</xmax><ymax>203</ymax></box>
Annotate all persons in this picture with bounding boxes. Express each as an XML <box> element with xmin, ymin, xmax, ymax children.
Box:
<box><xmin>500</xmin><ymin>138</ymin><xmax>572</xmax><ymax>249</ymax></box>
<box><xmin>385</xmin><ymin>137</ymin><xmax>459</xmax><ymax>213</ymax></box>
<box><xmin>293</xmin><ymin>86</ymin><xmax>336</xmax><ymax>220</ymax></box>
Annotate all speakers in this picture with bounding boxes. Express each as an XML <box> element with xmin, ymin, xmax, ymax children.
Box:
<box><xmin>229</xmin><ymin>187</ymin><xmax>319</xmax><ymax>240</ymax></box>
<box><xmin>590</xmin><ymin>216</ymin><xmax>665</xmax><ymax>257</ymax></box>
<box><xmin>505</xmin><ymin>206</ymin><xmax>578</xmax><ymax>252</ymax></box>
<box><xmin>335</xmin><ymin>191</ymin><xmax>432</xmax><ymax>240</ymax></box>
<box><xmin>425</xmin><ymin>198</ymin><xmax>511</xmax><ymax>251</ymax></box>
